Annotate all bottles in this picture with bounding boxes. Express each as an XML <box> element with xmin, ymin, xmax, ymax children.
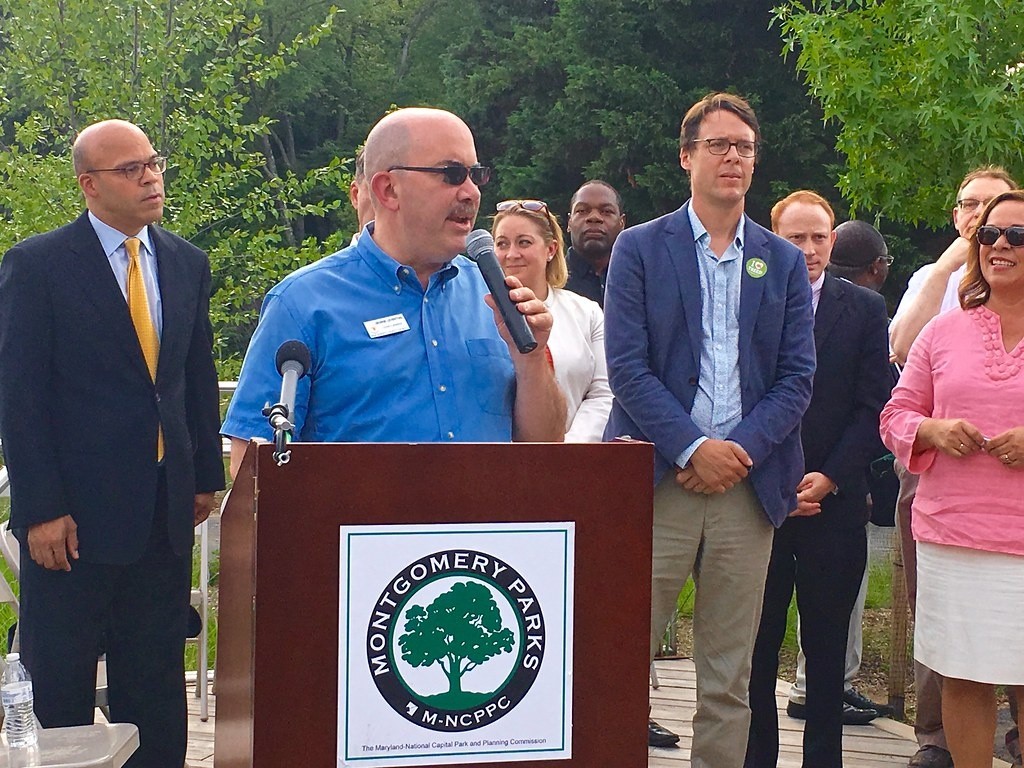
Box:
<box><xmin>0</xmin><ymin>653</ymin><xmax>38</xmax><ymax>749</ymax></box>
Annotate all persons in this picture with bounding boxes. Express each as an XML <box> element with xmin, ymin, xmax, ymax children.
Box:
<box><xmin>889</xmin><ymin>166</ymin><xmax>1024</xmax><ymax>768</ymax></box>
<box><xmin>349</xmin><ymin>147</ymin><xmax>374</xmax><ymax>246</ymax></box>
<box><xmin>602</xmin><ymin>91</ymin><xmax>815</xmax><ymax>768</ymax></box>
<box><xmin>562</xmin><ymin>179</ymin><xmax>680</xmax><ymax>749</ymax></box>
<box><xmin>0</xmin><ymin>119</ymin><xmax>226</xmax><ymax>768</ymax></box>
<box><xmin>879</xmin><ymin>191</ymin><xmax>1024</xmax><ymax>768</ymax></box>
<box><xmin>786</xmin><ymin>220</ymin><xmax>903</xmax><ymax>726</ymax></box>
<box><xmin>492</xmin><ymin>199</ymin><xmax>615</xmax><ymax>443</ymax></box>
<box><xmin>219</xmin><ymin>108</ymin><xmax>568</xmax><ymax>483</ymax></box>
<box><xmin>745</xmin><ymin>191</ymin><xmax>894</xmax><ymax>768</ymax></box>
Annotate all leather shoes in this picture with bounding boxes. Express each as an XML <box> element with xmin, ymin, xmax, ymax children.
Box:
<box><xmin>648</xmin><ymin>716</ymin><xmax>680</xmax><ymax>747</ymax></box>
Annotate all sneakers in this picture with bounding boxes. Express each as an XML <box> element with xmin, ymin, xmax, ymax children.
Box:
<box><xmin>786</xmin><ymin>696</ymin><xmax>877</xmax><ymax>724</ymax></box>
<box><xmin>842</xmin><ymin>686</ymin><xmax>894</xmax><ymax>716</ymax></box>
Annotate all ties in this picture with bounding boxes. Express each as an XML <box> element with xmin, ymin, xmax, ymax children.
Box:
<box><xmin>124</xmin><ymin>237</ymin><xmax>166</xmax><ymax>463</ymax></box>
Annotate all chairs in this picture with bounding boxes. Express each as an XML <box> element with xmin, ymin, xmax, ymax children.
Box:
<box><xmin>0</xmin><ymin>519</ymin><xmax>208</xmax><ymax>768</ymax></box>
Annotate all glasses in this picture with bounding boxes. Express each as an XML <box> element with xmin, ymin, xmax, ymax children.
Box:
<box><xmin>958</xmin><ymin>198</ymin><xmax>994</xmax><ymax>212</ymax></box>
<box><xmin>879</xmin><ymin>255</ymin><xmax>894</xmax><ymax>266</ymax></box>
<box><xmin>976</xmin><ymin>226</ymin><xmax>1024</xmax><ymax>248</ymax></box>
<box><xmin>88</xmin><ymin>156</ymin><xmax>168</xmax><ymax>181</ymax></box>
<box><xmin>692</xmin><ymin>138</ymin><xmax>760</xmax><ymax>157</ymax></box>
<box><xmin>496</xmin><ymin>199</ymin><xmax>551</xmax><ymax>226</ymax></box>
<box><xmin>388</xmin><ymin>160</ymin><xmax>491</xmax><ymax>187</ymax></box>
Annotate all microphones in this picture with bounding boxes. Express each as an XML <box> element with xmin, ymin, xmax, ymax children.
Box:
<box><xmin>465</xmin><ymin>229</ymin><xmax>538</xmax><ymax>354</ymax></box>
<box><xmin>276</xmin><ymin>340</ymin><xmax>311</xmax><ymax>443</ymax></box>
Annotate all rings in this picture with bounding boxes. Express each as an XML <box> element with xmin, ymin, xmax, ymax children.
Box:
<box><xmin>959</xmin><ymin>444</ymin><xmax>963</xmax><ymax>451</ymax></box>
<box><xmin>1005</xmin><ymin>454</ymin><xmax>1011</xmax><ymax>463</ymax></box>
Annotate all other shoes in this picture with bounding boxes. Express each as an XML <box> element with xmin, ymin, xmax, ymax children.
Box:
<box><xmin>907</xmin><ymin>745</ymin><xmax>954</xmax><ymax>768</ymax></box>
